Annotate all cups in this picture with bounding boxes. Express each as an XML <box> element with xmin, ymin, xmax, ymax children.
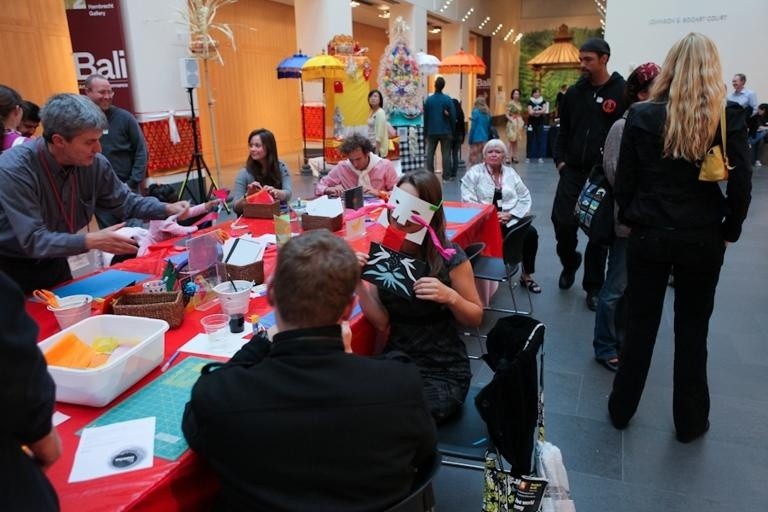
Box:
<box><xmin>212</xmin><ymin>279</ymin><xmax>252</xmax><ymax>318</ymax></box>
<box><xmin>200</xmin><ymin>314</ymin><xmax>231</xmax><ymax>344</ymax></box>
<box><xmin>227</xmin><ymin>314</ymin><xmax>244</xmax><ymax>334</ymax></box>
<box><xmin>47</xmin><ymin>294</ymin><xmax>93</xmax><ymax>331</ymax></box>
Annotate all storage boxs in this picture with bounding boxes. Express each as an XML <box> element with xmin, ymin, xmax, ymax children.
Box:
<box><xmin>39</xmin><ymin>313</ymin><xmax>170</xmax><ymax>407</ymax></box>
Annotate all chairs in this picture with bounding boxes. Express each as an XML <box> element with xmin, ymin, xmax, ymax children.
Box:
<box><xmin>385</xmin><ymin>453</ymin><xmax>443</xmax><ymax>512</ymax></box>
<box><xmin>470</xmin><ymin>215</ymin><xmax>537</xmax><ymax>316</ymax></box>
<box><xmin>464</xmin><ymin>242</ymin><xmax>486</xmax><ymax>360</ymax></box>
<box><xmin>437</xmin><ymin>323</ymin><xmax>546</xmax><ymax>475</ymax></box>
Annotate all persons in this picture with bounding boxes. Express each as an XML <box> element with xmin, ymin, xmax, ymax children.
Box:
<box><xmin>351</xmin><ymin>169</ymin><xmax>484</xmax><ymax>435</ymax></box>
<box><xmin>550</xmin><ymin>34</ymin><xmax>768</xmax><ymax>373</ymax></box>
<box><xmin>234</xmin><ymin>128</ymin><xmax>291</xmax><ymax>213</ymax></box>
<box><xmin>182</xmin><ymin>228</ymin><xmax>442</xmax><ymax>510</ymax></box>
<box><xmin>460</xmin><ymin>138</ymin><xmax>541</xmax><ymax>293</ymax></box>
<box><xmin>608</xmin><ymin>33</ymin><xmax>756</xmax><ymax>441</ymax></box>
<box><xmin>1</xmin><ymin>274</ymin><xmax>61</xmax><ymax>511</ymax></box>
<box><xmin>366</xmin><ymin>92</ymin><xmax>388</xmax><ymax>159</ymax></box>
<box><xmin>313</xmin><ymin>131</ymin><xmax>401</xmax><ymax>200</ymax></box>
<box><xmin>1</xmin><ymin>70</ymin><xmax>226</xmax><ymax>295</ymax></box>
<box><xmin>422</xmin><ymin>77</ymin><xmax>547</xmax><ymax>182</ymax></box>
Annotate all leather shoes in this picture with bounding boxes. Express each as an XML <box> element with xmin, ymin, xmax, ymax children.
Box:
<box><xmin>586</xmin><ymin>294</ymin><xmax>598</xmax><ymax>310</ymax></box>
<box><xmin>559</xmin><ymin>252</ymin><xmax>582</xmax><ymax>290</ymax></box>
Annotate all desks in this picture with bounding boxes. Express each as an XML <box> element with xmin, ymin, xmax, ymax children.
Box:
<box><xmin>22</xmin><ymin>201</ymin><xmax>502</xmax><ymax>512</ymax></box>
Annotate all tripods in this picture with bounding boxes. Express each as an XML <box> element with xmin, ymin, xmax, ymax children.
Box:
<box><xmin>178</xmin><ymin>90</ymin><xmax>232</xmax><ymax>215</ymax></box>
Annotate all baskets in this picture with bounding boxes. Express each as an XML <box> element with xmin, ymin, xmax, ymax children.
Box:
<box><xmin>112</xmin><ymin>289</ymin><xmax>184</xmax><ymax>329</ymax></box>
<box><xmin>242</xmin><ymin>199</ymin><xmax>280</xmax><ymax>220</ymax></box>
<box><xmin>301</xmin><ymin>212</ymin><xmax>342</xmax><ymax>231</ymax></box>
<box><xmin>215</xmin><ymin>253</ymin><xmax>264</xmax><ymax>285</ymax></box>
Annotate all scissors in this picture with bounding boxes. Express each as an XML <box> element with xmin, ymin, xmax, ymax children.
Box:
<box><xmin>32</xmin><ymin>289</ymin><xmax>61</xmax><ymax>309</ymax></box>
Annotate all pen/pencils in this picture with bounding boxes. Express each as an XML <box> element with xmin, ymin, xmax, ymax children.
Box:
<box><xmin>227</xmin><ymin>273</ymin><xmax>240</xmax><ymax>291</ymax></box>
<box><xmin>161</xmin><ymin>350</ymin><xmax>180</xmax><ymax>373</ymax></box>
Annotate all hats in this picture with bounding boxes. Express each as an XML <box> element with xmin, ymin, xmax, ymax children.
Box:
<box><xmin>624</xmin><ymin>63</ymin><xmax>661</xmax><ymax>92</ymax></box>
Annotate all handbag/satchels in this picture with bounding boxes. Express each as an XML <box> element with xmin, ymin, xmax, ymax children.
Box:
<box><xmin>574</xmin><ymin>160</ymin><xmax>614</xmax><ymax>241</ymax></box>
<box><xmin>488</xmin><ymin>123</ymin><xmax>500</xmax><ymax>139</ymax></box>
<box><xmin>483</xmin><ymin>440</ymin><xmax>549</xmax><ymax>512</ymax></box>
<box><xmin>699</xmin><ymin>146</ymin><xmax>729</xmax><ymax>183</ymax></box>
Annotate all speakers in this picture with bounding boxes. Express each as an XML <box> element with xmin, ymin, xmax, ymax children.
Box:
<box><xmin>181</xmin><ymin>57</ymin><xmax>200</xmax><ymax>89</ymax></box>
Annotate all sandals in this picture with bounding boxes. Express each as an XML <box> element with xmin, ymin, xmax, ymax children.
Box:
<box><xmin>520</xmin><ymin>276</ymin><xmax>541</xmax><ymax>293</ymax></box>
